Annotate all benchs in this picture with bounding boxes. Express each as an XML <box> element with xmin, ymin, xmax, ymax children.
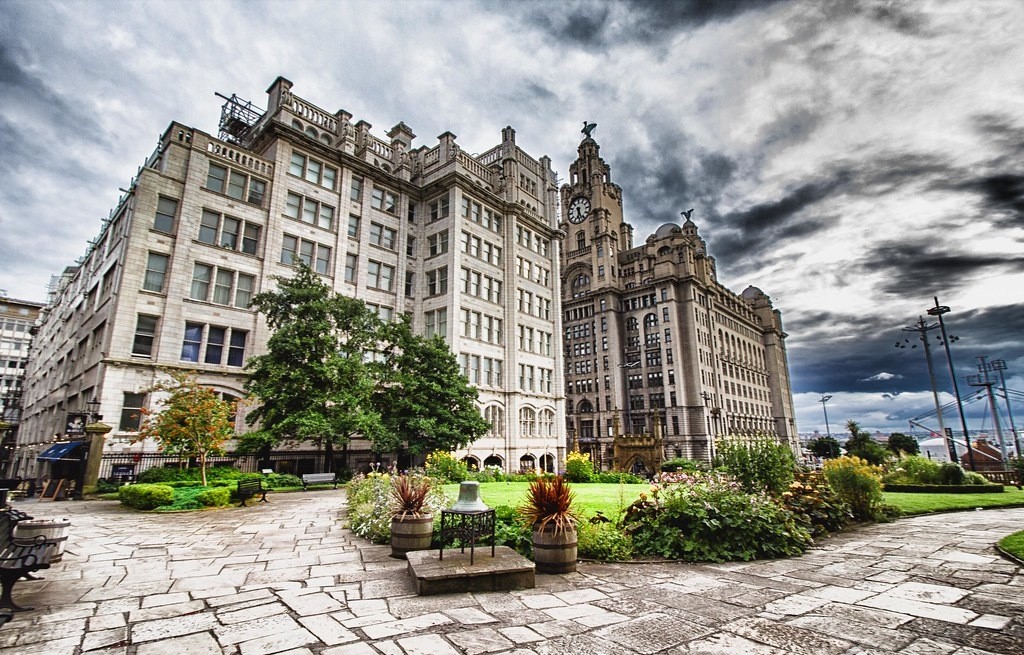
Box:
<box><xmin>302</xmin><ymin>472</ymin><xmax>339</xmax><ymax>492</ymax></box>
<box><xmin>0</xmin><ymin>503</ymin><xmax>57</xmax><ymax>614</ymax></box>
<box><xmin>238</xmin><ymin>477</ymin><xmax>274</xmax><ymax>507</ymax></box>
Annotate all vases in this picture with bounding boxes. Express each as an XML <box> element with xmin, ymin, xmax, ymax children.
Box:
<box><xmin>16</xmin><ymin>518</ymin><xmax>71</xmax><ymax>564</ymax></box>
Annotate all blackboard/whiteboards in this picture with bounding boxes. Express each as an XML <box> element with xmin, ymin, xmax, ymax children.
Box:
<box><xmin>41</xmin><ymin>479</ymin><xmax>65</xmax><ymax>498</ymax></box>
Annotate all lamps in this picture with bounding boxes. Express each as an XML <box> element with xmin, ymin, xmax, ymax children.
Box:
<box><xmin>54</xmin><ymin>433</ymin><xmax>61</xmax><ymax>439</ymax></box>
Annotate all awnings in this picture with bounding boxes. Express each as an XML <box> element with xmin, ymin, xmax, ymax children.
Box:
<box><xmin>37</xmin><ymin>442</ymin><xmax>87</xmax><ymax>462</ymax></box>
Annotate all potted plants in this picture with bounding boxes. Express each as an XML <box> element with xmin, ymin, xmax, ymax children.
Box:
<box><xmin>516</xmin><ymin>473</ymin><xmax>578</xmax><ymax>574</ymax></box>
<box><xmin>389</xmin><ymin>475</ymin><xmax>434</xmax><ymax>560</ymax></box>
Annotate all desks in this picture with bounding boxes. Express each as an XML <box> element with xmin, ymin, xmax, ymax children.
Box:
<box><xmin>439</xmin><ymin>508</ymin><xmax>495</xmax><ymax>565</ymax></box>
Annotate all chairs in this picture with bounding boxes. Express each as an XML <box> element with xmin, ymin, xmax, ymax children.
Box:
<box><xmin>7</xmin><ymin>481</ymin><xmax>30</xmax><ymax>502</ymax></box>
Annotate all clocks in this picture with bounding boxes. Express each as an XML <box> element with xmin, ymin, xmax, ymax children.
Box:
<box><xmin>567</xmin><ymin>196</ymin><xmax>591</xmax><ymax>224</ymax></box>
